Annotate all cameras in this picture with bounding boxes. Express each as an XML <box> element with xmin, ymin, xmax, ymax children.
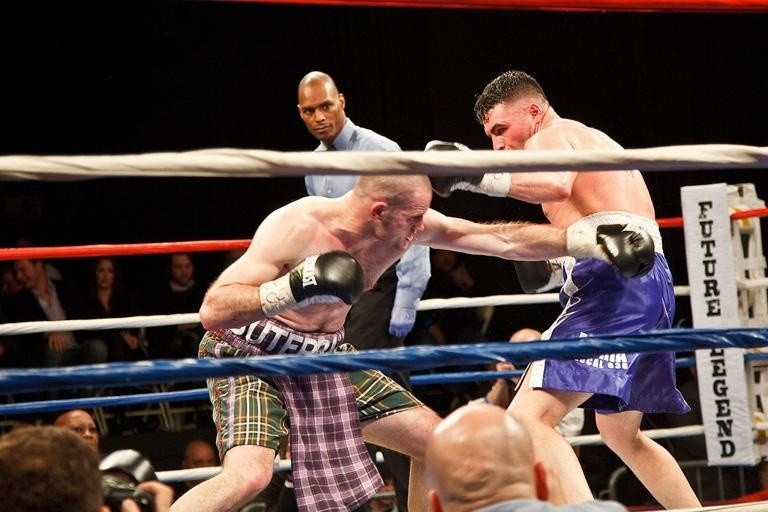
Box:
<box><xmin>102</xmin><ymin>479</ymin><xmax>153</xmax><ymax>512</ymax></box>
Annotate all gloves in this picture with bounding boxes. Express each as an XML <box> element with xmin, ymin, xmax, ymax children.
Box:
<box><xmin>515</xmin><ymin>255</ymin><xmax>567</xmax><ymax>297</ymax></box>
<box><xmin>257</xmin><ymin>251</ymin><xmax>365</xmax><ymax>324</ymax></box>
<box><xmin>562</xmin><ymin>218</ymin><xmax>657</xmax><ymax>280</ymax></box>
<box><xmin>386</xmin><ymin>307</ymin><xmax>418</xmax><ymax>339</ymax></box>
<box><xmin>422</xmin><ymin>138</ymin><xmax>514</xmax><ymax>200</ymax></box>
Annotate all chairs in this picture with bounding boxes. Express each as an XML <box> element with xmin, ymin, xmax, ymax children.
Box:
<box><xmin>1</xmin><ymin>325</ymin><xmax>216</xmax><ymax>441</ymax></box>
